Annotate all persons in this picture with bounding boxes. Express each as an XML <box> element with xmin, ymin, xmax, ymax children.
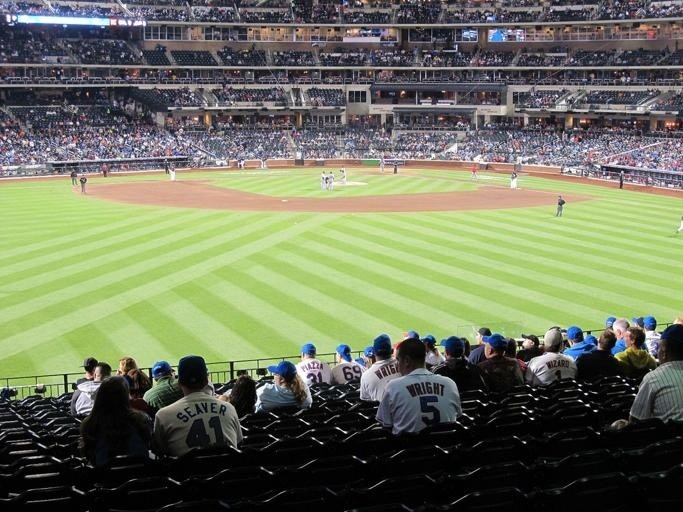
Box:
<box><xmin>80</xmin><ymin>174</ymin><xmax>86</xmax><ymax>194</ymax></box>
<box><xmin>1</xmin><ymin>0</ymin><xmax>683</xmax><ymax>189</ymax></box>
<box><xmin>556</xmin><ymin>194</ymin><xmax>565</xmax><ymax>217</ymax></box>
<box><xmin>68</xmin><ymin>313</ymin><xmax>682</xmax><ymax>467</ymax></box>
<box><xmin>677</xmin><ymin>216</ymin><xmax>683</xmax><ymax>233</ymax></box>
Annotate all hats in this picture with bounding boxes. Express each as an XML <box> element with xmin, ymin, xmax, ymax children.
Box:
<box><xmin>80</xmin><ymin>357</ymin><xmax>97</xmax><ymax>367</ymax></box>
<box><xmin>566</xmin><ymin>326</ymin><xmax>598</xmax><ymax>346</ymax></box>
<box><xmin>606</xmin><ymin>317</ymin><xmax>617</xmax><ymax>327</ymax></box>
<box><xmin>355</xmin><ymin>334</ymin><xmax>391</xmax><ymax>367</ymax></box>
<box><xmin>408</xmin><ymin>331</ymin><xmax>436</xmax><ymax>344</ymax></box>
<box><xmin>178</xmin><ymin>356</ymin><xmax>207</xmax><ymax>376</ymax></box>
<box><xmin>472</xmin><ymin>326</ymin><xmax>491</xmax><ymax>336</ymax></box>
<box><xmin>335</xmin><ymin>344</ymin><xmax>352</xmax><ymax>362</ymax></box>
<box><xmin>522</xmin><ymin>333</ymin><xmax>539</xmax><ymax>346</ymax></box>
<box><xmin>268</xmin><ymin>361</ymin><xmax>297</xmax><ymax>379</ymax></box>
<box><xmin>632</xmin><ymin>316</ymin><xmax>657</xmax><ymax>331</ymax></box>
<box><xmin>544</xmin><ymin>329</ymin><xmax>563</xmax><ymax>346</ymax></box>
<box><xmin>482</xmin><ymin>333</ymin><xmax>507</xmax><ymax>350</ymax></box>
<box><xmin>301</xmin><ymin>344</ymin><xmax>316</xmax><ymax>355</ymax></box>
<box><xmin>151</xmin><ymin>361</ymin><xmax>175</xmax><ymax>377</ymax></box>
<box><xmin>440</xmin><ymin>336</ymin><xmax>463</xmax><ymax>353</ymax></box>
